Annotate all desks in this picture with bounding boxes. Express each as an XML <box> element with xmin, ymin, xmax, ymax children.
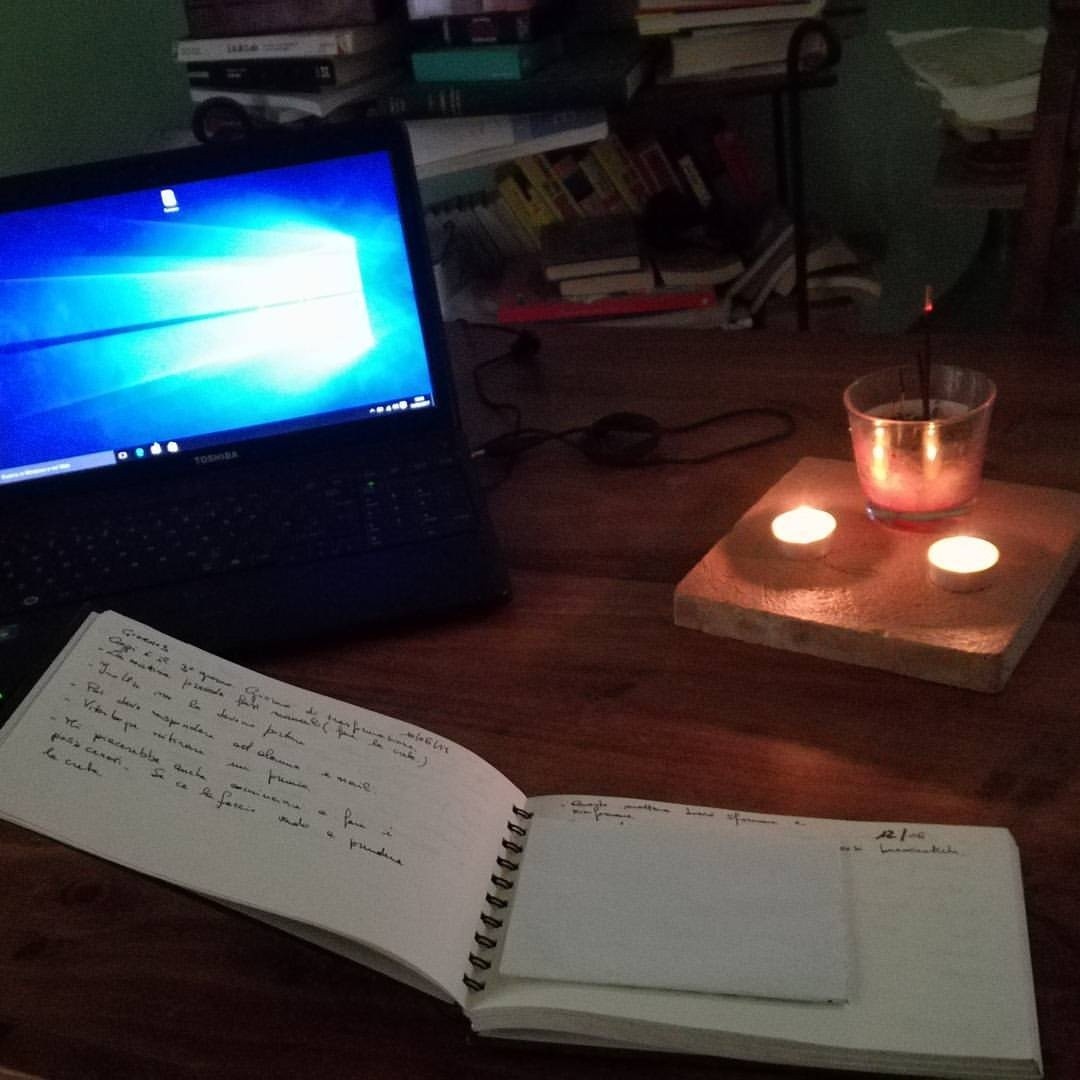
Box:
<box><xmin>632</xmin><ymin>21</ymin><xmax>843</xmax><ymax>330</ymax></box>
<box><xmin>1</xmin><ymin>318</ymin><xmax>1080</xmax><ymax>1080</ymax></box>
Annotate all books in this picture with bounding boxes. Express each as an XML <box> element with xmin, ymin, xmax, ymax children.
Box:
<box><xmin>171</xmin><ymin>0</ymin><xmax>883</xmax><ymax>328</ymax></box>
<box><xmin>0</xmin><ymin>609</ymin><xmax>1045</xmax><ymax>1080</ymax></box>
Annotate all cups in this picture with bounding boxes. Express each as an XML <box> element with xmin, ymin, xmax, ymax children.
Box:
<box><xmin>842</xmin><ymin>363</ymin><xmax>998</xmax><ymax>522</ymax></box>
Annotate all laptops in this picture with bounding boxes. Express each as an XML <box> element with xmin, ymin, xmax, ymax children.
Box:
<box><xmin>0</xmin><ymin>114</ymin><xmax>513</xmax><ymax>715</ymax></box>
